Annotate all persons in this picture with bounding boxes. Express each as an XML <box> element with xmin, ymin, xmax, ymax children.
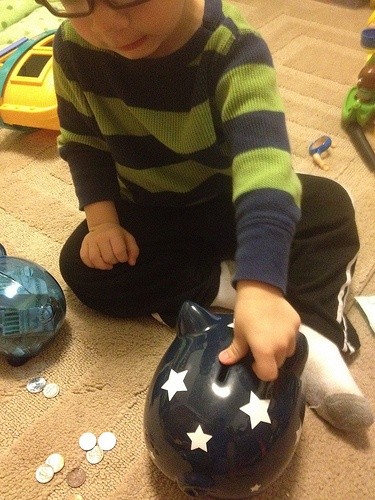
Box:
<box><xmin>35</xmin><ymin>0</ymin><xmax>374</xmax><ymax>432</ymax></box>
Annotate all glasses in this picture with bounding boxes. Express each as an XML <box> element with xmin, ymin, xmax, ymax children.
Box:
<box><xmin>34</xmin><ymin>1</ymin><xmax>153</xmax><ymax>18</ymax></box>
